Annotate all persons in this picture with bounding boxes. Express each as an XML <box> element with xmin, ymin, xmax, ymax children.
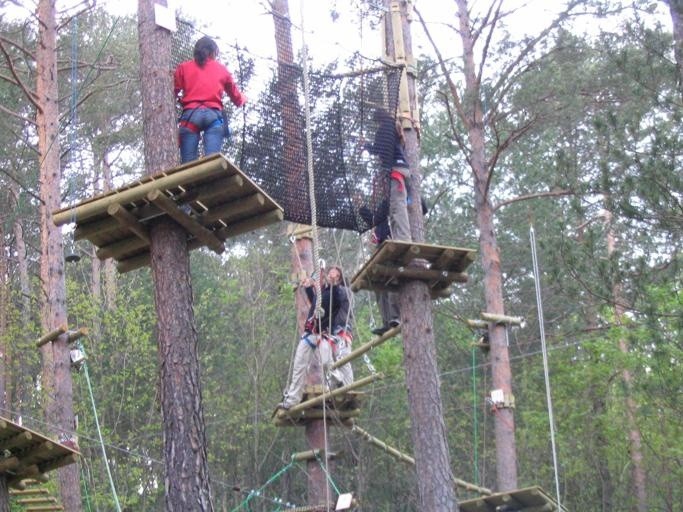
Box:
<box><xmin>173</xmin><ymin>36</ymin><xmax>247</xmax><ymax>164</ymax></box>
<box><xmin>355</xmin><ymin>192</ymin><xmax>400</xmax><ymax>336</ymax></box>
<box><xmin>361</xmin><ymin>109</ymin><xmax>408</xmax><ymax>240</ymax></box>
<box><xmin>282</xmin><ymin>266</ymin><xmax>350</xmax><ymax>409</ymax></box>
<box><xmin>332</xmin><ymin>277</ymin><xmax>354</xmax><ymax>389</ymax></box>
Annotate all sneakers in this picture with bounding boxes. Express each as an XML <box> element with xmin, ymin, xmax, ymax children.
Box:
<box><xmin>369</xmin><ymin>327</ymin><xmax>386</xmax><ymax>335</ymax></box>
<box><xmin>389</xmin><ymin>320</ymin><xmax>400</xmax><ymax>328</ymax></box>
